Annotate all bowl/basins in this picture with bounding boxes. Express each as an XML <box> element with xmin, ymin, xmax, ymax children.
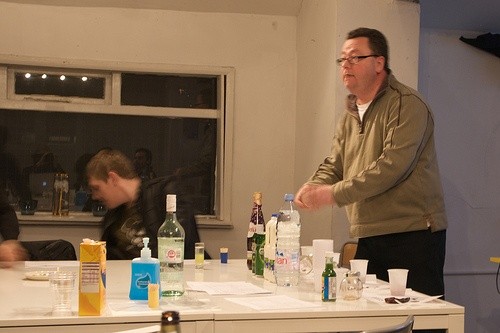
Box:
<box><xmin>19</xmin><ymin>200</ymin><xmax>37</xmax><ymax>215</ymax></box>
<box><xmin>92</xmin><ymin>200</ymin><xmax>106</xmax><ymax>217</ymax></box>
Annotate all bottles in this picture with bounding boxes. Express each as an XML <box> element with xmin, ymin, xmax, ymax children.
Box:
<box><xmin>321</xmin><ymin>256</ymin><xmax>337</xmax><ymax>303</ymax></box>
<box><xmin>246</xmin><ymin>192</ymin><xmax>263</xmax><ymax>270</ymax></box>
<box><xmin>263</xmin><ymin>213</ymin><xmax>278</xmax><ymax>283</ymax></box>
<box><xmin>157</xmin><ymin>194</ymin><xmax>184</xmax><ymax>296</ymax></box>
<box><xmin>251</xmin><ymin>224</ymin><xmax>265</xmax><ymax>275</ymax></box>
<box><xmin>274</xmin><ymin>194</ymin><xmax>300</xmax><ymax>288</ymax></box>
<box><xmin>161</xmin><ymin>312</ymin><xmax>181</xmax><ymax>333</ymax></box>
<box><xmin>340</xmin><ymin>272</ymin><xmax>363</xmax><ymax>300</ymax></box>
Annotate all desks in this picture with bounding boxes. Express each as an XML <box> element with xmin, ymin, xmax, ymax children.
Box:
<box><xmin>0</xmin><ymin>260</ymin><xmax>466</xmax><ymax>333</ymax></box>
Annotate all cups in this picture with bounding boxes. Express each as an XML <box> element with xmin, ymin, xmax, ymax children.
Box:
<box><xmin>220</xmin><ymin>248</ymin><xmax>227</xmax><ymax>263</ymax></box>
<box><xmin>301</xmin><ymin>246</ymin><xmax>314</xmax><ymax>283</ymax></box>
<box><xmin>349</xmin><ymin>259</ymin><xmax>368</xmax><ymax>284</ymax></box>
<box><xmin>195</xmin><ymin>243</ymin><xmax>204</xmax><ymax>269</ymax></box>
<box><xmin>388</xmin><ymin>269</ymin><xmax>410</xmax><ymax>296</ymax></box>
<box><xmin>52</xmin><ymin>272</ymin><xmax>76</xmax><ymax>310</ymax></box>
<box><xmin>311</xmin><ymin>239</ymin><xmax>334</xmax><ymax>293</ymax></box>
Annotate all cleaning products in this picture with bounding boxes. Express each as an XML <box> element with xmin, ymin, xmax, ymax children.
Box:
<box><xmin>128</xmin><ymin>236</ymin><xmax>162</xmax><ymax>300</ymax></box>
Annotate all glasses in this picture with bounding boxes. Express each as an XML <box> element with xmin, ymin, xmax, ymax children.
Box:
<box><xmin>336</xmin><ymin>55</ymin><xmax>381</xmax><ymax>67</ymax></box>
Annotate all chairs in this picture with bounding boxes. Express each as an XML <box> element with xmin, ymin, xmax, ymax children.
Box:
<box><xmin>18</xmin><ymin>239</ymin><xmax>78</xmax><ymax>260</ymax></box>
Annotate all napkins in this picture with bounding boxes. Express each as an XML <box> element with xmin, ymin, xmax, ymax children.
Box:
<box><xmin>365</xmin><ymin>275</ymin><xmax>377</xmax><ymax>284</ymax></box>
<box><xmin>187</xmin><ymin>281</ymin><xmax>272</xmax><ymax>295</ymax></box>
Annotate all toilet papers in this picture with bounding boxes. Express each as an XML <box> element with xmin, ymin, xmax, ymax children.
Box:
<box><xmin>312</xmin><ymin>238</ymin><xmax>334</xmax><ymax>292</ymax></box>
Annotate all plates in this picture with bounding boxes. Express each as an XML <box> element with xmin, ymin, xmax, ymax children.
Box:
<box><xmin>24</xmin><ymin>271</ymin><xmax>52</xmax><ymax>281</ymax></box>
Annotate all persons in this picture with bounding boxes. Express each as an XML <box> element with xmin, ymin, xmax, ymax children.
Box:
<box><xmin>85</xmin><ymin>148</ymin><xmax>211</xmax><ymax>260</ymax></box>
<box><xmin>0</xmin><ymin>199</ymin><xmax>32</xmax><ymax>260</ymax></box>
<box><xmin>28</xmin><ymin>147</ymin><xmax>160</xmax><ymax>205</ymax></box>
<box><xmin>294</xmin><ymin>28</ymin><xmax>447</xmax><ymax>333</ymax></box>
